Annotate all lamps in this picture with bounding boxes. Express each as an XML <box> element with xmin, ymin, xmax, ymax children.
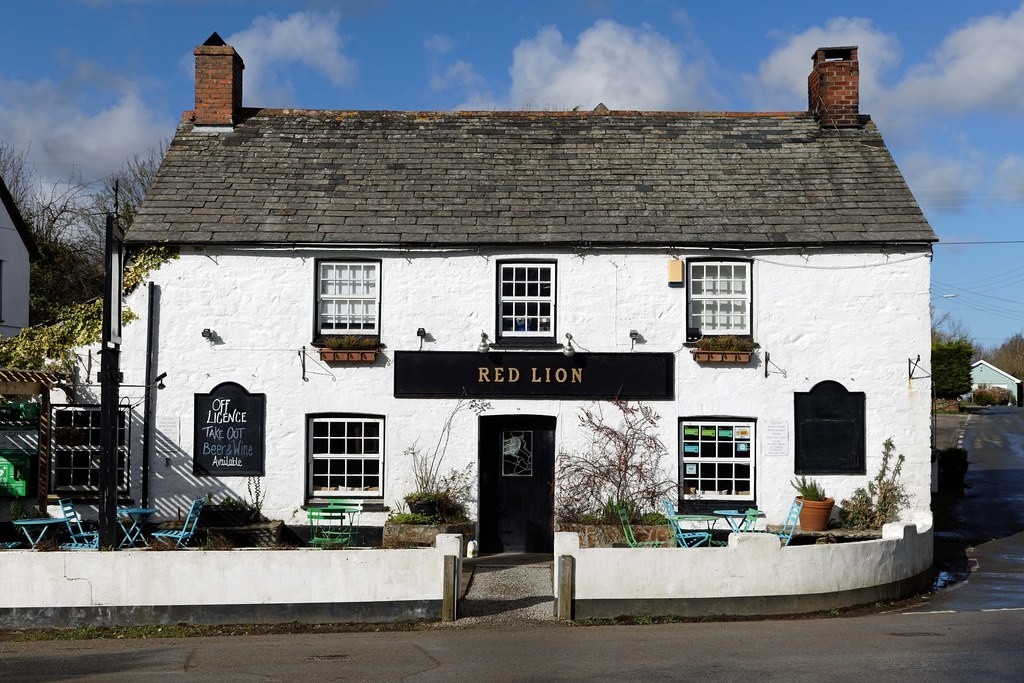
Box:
<box><xmin>629</xmin><ymin>330</ymin><xmax>638</xmax><ymax>338</ymax></box>
<box><xmin>563</xmin><ymin>333</ymin><xmax>577</xmax><ymax>356</ymax></box>
<box><xmin>202</xmin><ymin>329</ymin><xmax>212</xmax><ymax>337</ymax></box>
<box><xmin>417</xmin><ymin>328</ymin><xmax>425</xmax><ymax>336</ymax></box>
<box><xmin>478</xmin><ymin>333</ymin><xmax>490</xmax><ymax>353</ymax></box>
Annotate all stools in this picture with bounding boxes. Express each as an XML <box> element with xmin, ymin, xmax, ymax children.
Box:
<box><xmin>0</xmin><ymin>542</ymin><xmax>23</xmax><ymax>550</ymax></box>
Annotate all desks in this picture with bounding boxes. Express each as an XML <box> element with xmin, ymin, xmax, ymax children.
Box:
<box><xmin>670</xmin><ymin>515</ymin><xmax>718</xmax><ymax>534</ymax></box>
<box><xmin>10</xmin><ymin>519</ymin><xmax>68</xmax><ymax>548</ymax></box>
<box><xmin>714</xmin><ymin>509</ymin><xmax>763</xmax><ymax>534</ymax></box>
<box><xmin>308</xmin><ymin>507</ymin><xmax>359</xmax><ymax>546</ymax></box>
<box><xmin>116</xmin><ymin>507</ymin><xmax>158</xmax><ymax>550</ymax></box>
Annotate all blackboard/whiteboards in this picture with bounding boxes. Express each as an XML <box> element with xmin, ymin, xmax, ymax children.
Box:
<box><xmin>193</xmin><ymin>381</ymin><xmax>266</xmax><ymax>476</ymax></box>
<box><xmin>794</xmin><ymin>380</ymin><xmax>867</xmax><ymax>475</ymax></box>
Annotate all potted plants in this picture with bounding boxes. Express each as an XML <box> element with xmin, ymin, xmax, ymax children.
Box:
<box><xmin>403</xmin><ymin>492</ymin><xmax>444</xmax><ymax>517</ymax></box>
<box><xmin>319</xmin><ymin>337</ymin><xmax>379</xmax><ymax>361</ymax></box>
<box><xmin>936</xmin><ymin>447</ymin><xmax>968</xmax><ymax>497</ymax></box>
<box><xmin>790</xmin><ymin>473</ymin><xmax>834</xmax><ymax>531</ymax></box>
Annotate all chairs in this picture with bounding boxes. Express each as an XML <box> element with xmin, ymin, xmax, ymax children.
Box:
<box><xmin>617</xmin><ymin>501</ymin><xmax>759</xmax><ymax>548</ymax></box>
<box><xmin>307</xmin><ymin>498</ymin><xmax>364</xmax><ymax>549</ymax></box>
<box><xmin>56</xmin><ymin>498</ymin><xmax>101</xmax><ymax>551</ymax></box>
<box><xmin>151</xmin><ymin>498</ymin><xmax>205</xmax><ymax>550</ymax></box>
<box><xmin>760</xmin><ymin>500</ymin><xmax>802</xmax><ymax>546</ymax></box>
